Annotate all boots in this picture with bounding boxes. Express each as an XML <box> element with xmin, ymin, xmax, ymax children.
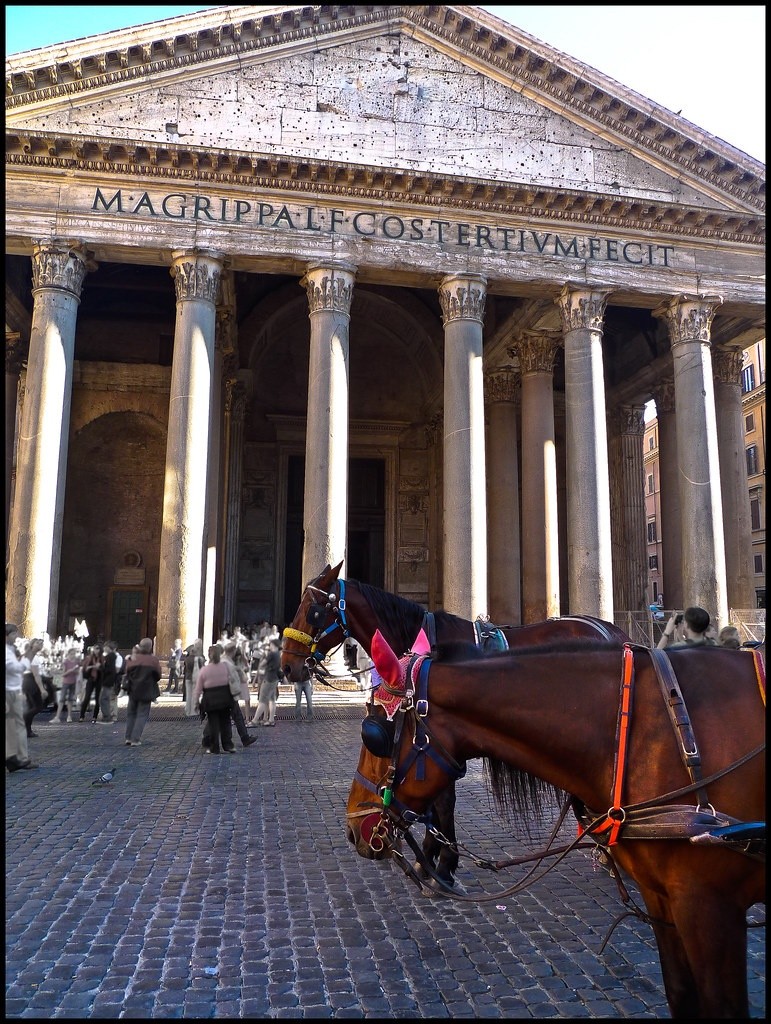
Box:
<box><xmin>6</xmin><ymin>755</ymin><xmax>31</xmax><ymax>771</ymax></box>
<box><xmin>25</xmin><ymin>760</ymin><xmax>41</xmax><ymax>768</ymax></box>
<box><xmin>242</xmin><ymin>735</ymin><xmax>257</xmax><ymax>746</ymax></box>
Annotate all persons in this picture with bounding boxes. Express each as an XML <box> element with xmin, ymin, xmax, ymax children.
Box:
<box><xmin>214</xmin><ymin>642</ymin><xmax>259</xmax><ymax>749</ymax></box>
<box><xmin>22</xmin><ymin>636</ymin><xmax>49</xmax><ymax>738</ymax></box>
<box><xmin>96</xmin><ymin>643</ymin><xmax>117</xmax><ymax>724</ymax></box>
<box><xmin>80</xmin><ymin>643</ymin><xmax>103</xmax><ymax>722</ymax></box>
<box><xmin>703</xmin><ymin>622</ymin><xmax>715</xmax><ymax>641</ymax></box>
<box><xmin>657</xmin><ymin>607</ymin><xmax>717</xmax><ymax>653</ymax></box>
<box><xmin>193</xmin><ymin>646</ymin><xmax>236</xmax><ymax>754</ymax></box>
<box><xmin>120</xmin><ymin>638</ymin><xmax>162</xmax><ymax>748</ymax></box>
<box><xmin>49</xmin><ymin>647</ymin><xmax>81</xmax><ymax>725</ymax></box>
<box><xmin>716</xmin><ymin>627</ymin><xmax>742</xmax><ymax>649</ymax></box>
<box><xmin>105</xmin><ymin>642</ymin><xmax>123</xmax><ymax>721</ymax></box>
<box><xmin>5</xmin><ymin>623</ymin><xmax>44</xmax><ymax>773</ymax></box>
<box><xmin>165</xmin><ymin>616</ymin><xmax>320</xmax><ymax>728</ymax></box>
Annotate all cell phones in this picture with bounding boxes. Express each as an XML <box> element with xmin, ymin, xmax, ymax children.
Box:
<box><xmin>675</xmin><ymin>613</ymin><xmax>683</xmax><ymax>625</ymax></box>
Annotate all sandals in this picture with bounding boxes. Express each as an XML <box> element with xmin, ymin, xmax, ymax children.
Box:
<box><xmin>245</xmin><ymin>721</ymin><xmax>258</xmax><ymax>728</ymax></box>
<box><xmin>263</xmin><ymin>719</ymin><xmax>276</xmax><ymax>726</ymax></box>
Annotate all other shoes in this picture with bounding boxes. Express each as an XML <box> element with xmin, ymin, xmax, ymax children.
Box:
<box><xmin>91</xmin><ymin>718</ymin><xmax>96</xmax><ymax>723</ymax></box>
<box><xmin>49</xmin><ymin>717</ymin><xmax>61</xmax><ymax>723</ymax></box>
<box><xmin>79</xmin><ymin>718</ymin><xmax>84</xmax><ymax>722</ymax></box>
<box><xmin>125</xmin><ymin>738</ymin><xmax>141</xmax><ymax>747</ymax></box>
<box><xmin>293</xmin><ymin>719</ymin><xmax>315</xmax><ymax>723</ymax></box>
<box><xmin>224</xmin><ymin>747</ymin><xmax>238</xmax><ymax>752</ymax></box>
<box><xmin>67</xmin><ymin>715</ymin><xmax>73</xmax><ymax>722</ymax></box>
<box><xmin>28</xmin><ymin>732</ymin><xmax>38</xmax><ymax>737</ymax></box>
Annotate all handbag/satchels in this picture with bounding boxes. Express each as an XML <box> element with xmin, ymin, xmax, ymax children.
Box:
<box><xmin>82</xmin><ymin>660</ymin><xmax>94</xmax><ymax>679</ymax></box>
<box><xmin>166</xmin><ymin>656</ymin><xmax>178</xmax><ymax>668</ymax></box>
<box><xmin>199</xmin><ymin>697</ymin><xmax>207</xmax><ymax>723</ymax></box>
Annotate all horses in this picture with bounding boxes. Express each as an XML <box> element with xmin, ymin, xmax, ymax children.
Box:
<box><xmin>345</xmin><ymin>626</ymin><xmax>767</xmax><ymax>1022</ymax></box>
<box><xmin>278</xmin><ymin>560</ymin><xmax>634</xmax><ymax>896</ymax></box>
